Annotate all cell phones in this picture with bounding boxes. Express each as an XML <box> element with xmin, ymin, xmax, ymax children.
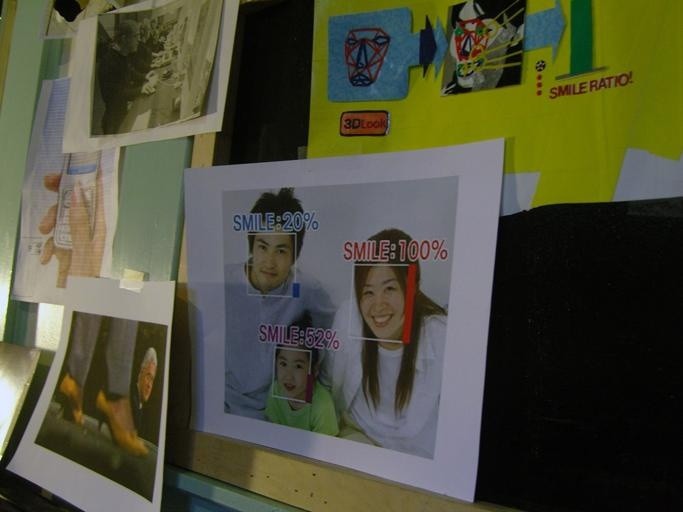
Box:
<box><xmin>53</xmin><ymin>149</ymin><xmax>102</xmax><ymax>252</ymax></box>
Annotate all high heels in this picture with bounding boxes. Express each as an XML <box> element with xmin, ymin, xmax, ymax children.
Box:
<box><xmin>55</xmin><ymin>375</ymin><xmax>149</xmax><ymax>456</ymax></box>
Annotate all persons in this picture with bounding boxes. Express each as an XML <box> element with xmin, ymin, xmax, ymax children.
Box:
<box><xmin>226</xmin><ymin>189</ymin><xmax>446</xmax><ymax>458</ymax></box>
<box><xmin>58</xmin><ymin>312</ymin><xmax>156</xmax><ymax>500</ymax></box>
<box><xmin>99</xmin><ymin>12</ymin><xmax>176</xmax><ymax>134</ymax></box>
